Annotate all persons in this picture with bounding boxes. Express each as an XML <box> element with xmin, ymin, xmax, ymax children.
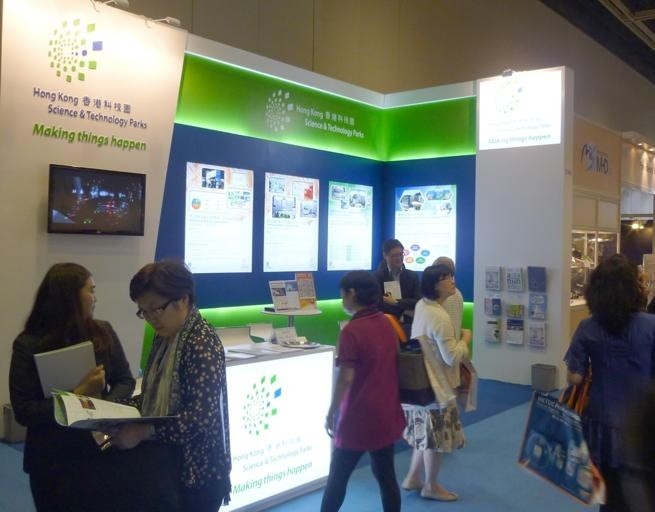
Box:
<box><xmin>563</xmin><ymin>253</ymin><xmax>655</xmax><ymax>512</ymax></box>
<box><xmin>9</xmin><ymin>262</ymin><xmax>137</xmax><ymax>512</ymax></box>
<box><xmin>399</xmin><ymin>266</ymin><xmax>467</xmax><ymax>501</ymax></box>
<box><xmin>110</xmin><ymin>261</ymin><xmax>232</xmax><ymax>512</ymax></box>
<box><xmin>431</xmin><ymin>256</ymin><xmax>464</xmax><ymax>343</ymax></box>
<box><xmin>372</xmin><ymin>239</ymin><xmax>420</xmax><ymax>326</ymax></box>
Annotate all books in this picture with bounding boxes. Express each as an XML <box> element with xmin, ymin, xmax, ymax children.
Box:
<box><xmin>506</xmin><ymin>267</ymin><xmax>523</xmax><ymax>292</ymax></box>
<box><xmin>483</xmin><ymin>297</ymin><xmax>501</xmax><ymax>317</ymax></box>
<box><xmin>506</xmin><ymin>320</ymin><xmax>524</xmax><ymax>344</ymax></box>
<box><xmin>320</xmin><ymin>270</ymin><xmax>409</xmax><ymax>512</ymax></box>
<box><xmin>485</xmin><ymin>266</ymin><xmax>501</xmax><ymax>291</ymax></box>
<box><xmin>527</xmin><ymin>322</ymin><xmax>545</xmax><ymax>347</ymax></box>
<box><xmin>506</xmin><ymin>304</ymin><xmax>524</xmax><ymax>316</ymax></box>
<box><xmin>384</xmin><ymin>280</ymin><xmax>402</xmax><ymax>301</ymax></box>
<box><xmin>527</xmin><ymin>266</ymin><xmax>546</xmax><ymax>292</ymax></box>
<box><xmin>34</xmin><ymin>341</ymin><xmax>97</xmax><ymax>398</ymax></box>
<box><xmin>482</xmin><ymin>320</ymin><xmax>501</xmax><ymax>343</ymax></box>
<box><xmin>50</xmin><ymin>389</ymin><xmax>179</xmax><ymax>430</ymax></box>
<box><xmin>528</xmin><ymin>293</ymin><xmax>546</xmax><ymax>320</ymax></box>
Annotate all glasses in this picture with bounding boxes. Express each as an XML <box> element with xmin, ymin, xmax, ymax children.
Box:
<box><xmin>384</xmin><ymin>251</ymin><xmax>405</xmax><ymax>259</ymax></box>
<box><xmin>435</xmin><ymin>276</ymin><xmax>455</xmax><ymax>282</ymax></box>
<box><xmin>136</xmin><ymin>296</ymin><xmax>182</xmax><ymax>320</ymax></box>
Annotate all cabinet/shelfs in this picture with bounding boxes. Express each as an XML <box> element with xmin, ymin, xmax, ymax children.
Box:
<box><xmin>225</xmin><ymin>338</ymin><xmax>337</xmax><ymax>512</ymax></box>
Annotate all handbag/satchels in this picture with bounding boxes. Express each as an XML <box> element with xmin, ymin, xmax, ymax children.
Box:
<box><xmin>457</xmin><ymin>355</ymin><xmax>477</xmax><ymax>413</ymax></box>
<box><xmin>515</xmin><ymin>378</ymin><xmax>609</xmax><ymax>506</ymax></box>
<box><xmin>384</xmin><ymin>312</ymin><xmax>436</xmax><ymax>407</ymax></box>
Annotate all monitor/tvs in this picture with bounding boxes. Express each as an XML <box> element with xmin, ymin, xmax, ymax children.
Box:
<box><xmin>47</xmin><ymin>163</ymin><xmax>148</xmax><ymax>237</ymax></box>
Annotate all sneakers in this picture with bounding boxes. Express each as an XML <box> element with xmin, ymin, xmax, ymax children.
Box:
<box><xmin>401</xmin><ymin>477</ymin><xmax>426</xmax><ymax>491</ymax></box>
<box><xmin>421</xmin><ymin>490</ymin><xmax>460</xmax><ymax>503</ymax></box>
